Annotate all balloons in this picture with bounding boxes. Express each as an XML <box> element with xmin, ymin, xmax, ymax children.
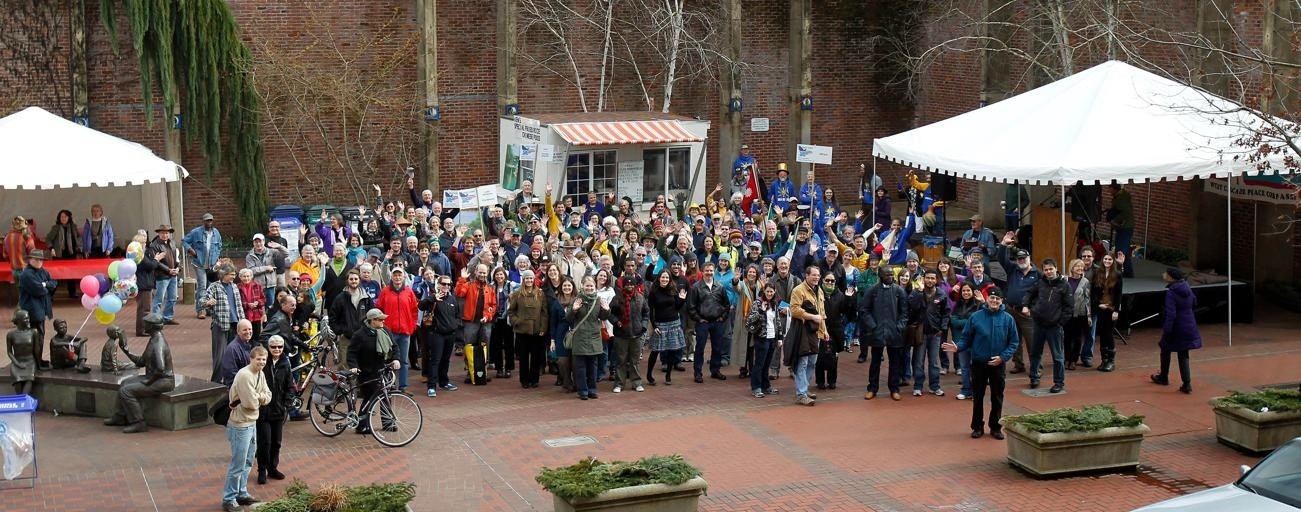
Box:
<box><xmin>79</xmin><ymin>275</ymin><xmax>100</xmax><ymax>298</ymax></box>
<box><xmin>98</xmin><ymin>292</ymin><xmax>122</xmax><ymax>314</ymax></box>
<box><xmin>95</xmin><ymin>308</ymin><xmax>116</xmax><ymax>325</ymax></box>
<box><xmin>81</xmin><ymin>293</ymin><xmax>101</xmax><ymax>310</ymax></box>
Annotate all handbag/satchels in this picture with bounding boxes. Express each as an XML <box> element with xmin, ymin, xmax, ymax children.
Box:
<box><xmin>600</xmin><ymin>321</ymin><xmax>609</xmax><ymax>341</ymax></box>
<box><xmin>209</xmin><ymin>393</ymin><xmax>231</xmax><ymax>426</ymax></box>
<box><xmin>562</xmin><ymin>329</ymin><xmax>573</xmax><ymax>350</ymax></box>
<box><xmin>91</xmin><ymin>238</ymin><xmax>98</xmax><ymax>250</ymax></box>
<box><xmin>422</xmin><ymin>301</ymin><xmax>436</xmax><ymax>328</ymax></box>
<box><xmin>311</xmin><ymin>370</ymin><xmax>340</xmax><ymax>406</ymax></box>
<box><xmin>904</xmin><ymin>321</ymin><xmax>924</xmax><ymax>346</ymax></box>
<box><xmin>826</xmin><ymin>319</ymin><xmax>845</xmax><ymax>353</ymax></box>
<box><xmin>745</xmin><ymin>297</ymin><xmax>765</xmax><ymax>335</ymax></box>
<box><xmin>463</xmin><ymin>321</ymin><xmax>489</xmax><ymax>385</ymax></box>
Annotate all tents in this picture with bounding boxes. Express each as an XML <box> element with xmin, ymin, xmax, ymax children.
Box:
<box><xmin>874</xmin><ymin>61</ymin><xmax>1301</xmax><ymax>346</ymax></box>
<box><xmin>0</xmin><ymin>107</ymin><xmax>189</xmax><ymax>295</ymax></box>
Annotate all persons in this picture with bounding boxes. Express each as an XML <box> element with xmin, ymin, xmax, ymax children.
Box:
<box><xmin>939</xmin><ymin>212</ymin><xmax>1125</xmax><ymax>440</ymax></box>
<box><xmin>6</xmin><ymin>311</ymin><xmax>176</xmax><ymax>432</ymax></box>
<box><xmin>1148</xmin><ymin>267</ymin><xmax>1202</xmax><ymax>394</ymax></box>
<box><xmin>1</xmin><ymin>204</ymin><xmax>180</xmax><ymax>336</ymax></box>
<box><xmin>256</xmin><ymin>334</ymin><xmax>294</xmax><ymax>485</ymax></box>
<box><xmin>1103</xmin><ymin>183</ymin><xmax>1135</xmax><ymax>279</ymax></box>
<box><xmin>202</xmin><ymin>177</ymin><xmax>463</xmax><ymax>421</ymax></box>
<box><xmin>448</xmin><ymin>179</ymin><xmax>732</xmax><ymax>399</ymax></box>
<box><xmin>346</xmin><ymin>308</ymin><xmax>402</xmax><ymax>433</ymax></box>
<box><xmin>711</xmin><ymin>143</ymin><xmax>953</xmax><ymax>407</ymax></box>
<box><xmin>222</xmin><ymin>345</ymin><xmax>272</xmax><ymax>509</ymax></box>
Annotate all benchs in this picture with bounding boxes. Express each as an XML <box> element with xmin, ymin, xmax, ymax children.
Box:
<box><xmin>0</xmin><ymin>361</ymin><xmax>229</xmax><ymax>433</ymax></box>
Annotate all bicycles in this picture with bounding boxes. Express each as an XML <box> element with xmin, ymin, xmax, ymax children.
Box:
<box><xmin>282</xmin><ymin>317</ymin><xmax>340</xmax><ymax>424</ymax></box>
<box><xmin>306</xmin><ymin>360</ymin><xmax>424</xmax><ymax>448</ymax></box>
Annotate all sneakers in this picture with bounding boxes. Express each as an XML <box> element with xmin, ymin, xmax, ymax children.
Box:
<box><xmin>612</xmin><ymin>387</ymin><xmax>621</xmax><ymax>393</ymax></box>
<box><xmin>223</xmin><ymin>467</ymin><xmax>285</xmax><ymax>512</ymax></box>
<box><xmin>427</xmin><ymin>389</ymin><xmax>436</xmax><ymax>397</ymax></box>
<box><xmin>846</xmin><ymin>346</ymin><xmax>853</xmax><ymax>353</ymax></box>
<box><xmin>853</xmin><ymin>339</ymin><xmax>860</xmax><ymax>346</ymax></box>
<box><xmin>635</xmin><ymin>386</ymin><xmax>644</xmax><ymax>392</ymax></box>
<box><xmin>454</xmin><ymin>347</ymin><xmax>462</xmax><ymax>356</ymax></box>
<box><xmin>806</xmin><ymin>392</ymin><xmax>816</xmax><ymax>398</ymax></box>
<box><xmin>646</xmin><ymin>352</ymin><xmax>779</xmax><ymax>398</ymax></box>
<box><xmin>438</xmin><ymin>383</ymin><xmax>457</xmax><ymax>391</ymax></box>
<box><xmin>794</xmin><ymin>394</ymin><xmax>816</xmax><ymax>406</ymax></box>
<box><xmin>1151</xmin><ymin>374</ymin><xmax>1168</xmax><ymax>384</ymax></box>
<box><xmin>912</xmin><ymin>368</ymin><xmax>972</xmax><ymax>400</ymax></box>
<box><xmin>1179</xmin><ymin>383</ymin><xmax>1192</xmax><ymax>392</ymax></box>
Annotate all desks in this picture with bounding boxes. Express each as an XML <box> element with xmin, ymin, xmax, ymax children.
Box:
<box><xmin>0</xmin><ymin>257</ymin><xmax>126</xmax><ymax>309</ymax></box>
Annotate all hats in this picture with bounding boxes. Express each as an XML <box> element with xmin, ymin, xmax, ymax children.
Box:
<box><xmin>684</xmin><ymin>203</ymin><xmax>761</xmax><ymax>262</ymax></box>
<box><xmin>825</xmin><ymin>243</ymin><xmax>838</xmax><ymax>255</ymax></box>
<box><xmin>788</xmin><ymin>196</ymin><xmax>799</xmax><ymax>203</ymax></box>
<box><xmin>407</xmin><ymin>166</ymin><xmax>415</xmax><ymax>178</ymax></box>
<box><xmin>510</xmin><ymin>198</ymin><xmax>541</xmax><ymax>237</ymax></box>
<box><xmin>740</xmin><ymin>143</ymin><xmax>749</xmax><ymax>149</ymax></box>
<box><xmin>905</xmin><ymin>251</ymin><xmax>918</xmax><ymax>262</ymax></box>
<box><xmin>155</xmin><ymin>224</ymin><xmax>175</xmax><ymax>233</ymax></box>
<box><xmin>875</xmin><ymin>185</ymin><xmax>888</xmax><ymax>194</ymax></box>
<box><xmin>299</xmin><ymin>273</ymin><xmax>312</xmax><ymax>284</ymax></box>
<box><xmin>653</xmin><ymin>221</ymin><xmax>662</xmax><ymax>229</ymax></box>
<box><xmin>1015</xmin><ymin>249</ymin><xmax>1029</xmax><ymax>259</ymax></box>
<box><xmin>798</xmin><ymin>226</ymin><xmax>809</xmax><ymax>233</ymax></box>
<box><xmin>969</xmin><ymin>214</ymin><xmax>982</xmax><ymax>221</ymax></box>
<box><xmin>641</xmin><ymin>234</ymin><xmax>657</xmax><ymax>248</ymax></box>
<box><xmin>570</xmin><ymin>209</ymin><xmax>581</xmax><ymax>215</ymax></box>
<box><xmin>202</xmin><ymin>213</ymin><xmax>213</xmax><ymax>221</ymax></box>
<box><xmin>776</xmin><ymin>162</ymin><xmax>789</xmax><ymax>175</ymax></box>
<box><xmin>25</xmin><ymin>249</ymin><xmax>48</xmax><ymax>261</ymax></box>
<box><xmin>392</xmin><ymin>216</ymin><xmax>412</xmax><ymax>228</ymax></box>
<box><xmin>366</xmin><ymin>308</ymin><xmax>390</xmax><ymax>320</ymax></box>
<box><xmin>368</xmin><ymin>247</ymin><xmax>381</xmax><ymax>259</ymax></box>
<box><xmin>622</xmin><ymin>275</ymin><xmax>636</xmax><ymax>288</ymax></box>
<box><xmin>530</xmin><ymin>239</ymin><xmax>576</xmax><ymax>263</ymax></box>
<box><xmin>987</xmin><ymin>286</ymin><xmax>1003</xmax><ymax>297</ymax></box>
<box><xmin>392</xmin><ymin>267</ymin><xmax>402</xmax><ymax>274</ymax></box>
<box><xmin>252</xmin><ymin>233</ymin><xmax>264</xmax><ymax>240</ymax></box>
<box><xmin>670</xmin><ymin>254</ymin><xmax>682</xmax><ymax>267</ymax></box>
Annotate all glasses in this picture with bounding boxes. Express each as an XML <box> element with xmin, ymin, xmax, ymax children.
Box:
<box><xmin>270</xmin><ymin>346</ymin><xmax>283</xmax><ymax>349</ymax></box>
<box><xmin>441</xmin><ymin>283</ymin><xmax>451</xmax><ymax>286</ymax></box>
<box><xmin>825</xmin><ymin>279</ymin><xmax>836</xmax><ymax>283</ymax></box>
<box><xmin>474</xmin><ymin>235</ymin><xmax>481</xmax><ymax>237</ymax></box>
<box><xmin>1082</xmin><ymin>255</ymin><xmax>1091</xmax><ymax>257</ymax></box>
<box><xmin>292</xmin><ymin>278</ymin><xmax>300</xmax><ymax>280</ymax></box>
<box><xmin>636</xmin><ymin>253</ymin><xmax>645</xmax><ymax>257</ymax></box>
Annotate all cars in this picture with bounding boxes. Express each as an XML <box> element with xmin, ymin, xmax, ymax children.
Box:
<box><xmin>1128</xmin><ymin>434</ymin><xmax>1301</xmax><ymax>512</ymax></box>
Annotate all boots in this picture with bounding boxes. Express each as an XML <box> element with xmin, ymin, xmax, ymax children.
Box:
<box><xmin>1097</xmin><ymin>347</ymin><xmax>1108</xmax><ymax>370</ymax></box>
<box><xmin>1102</xmin><ymin>348</ymin><xmax>1116</xmax><ymax>372</ymax></box>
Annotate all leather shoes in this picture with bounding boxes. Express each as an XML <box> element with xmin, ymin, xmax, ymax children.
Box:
<box><xmin>197</xmin><ymin>310</ymin><xmax>206</xmax><ymax>319</ymax></box>
<box><xmin>818</xmin><ymin>383</ymin><xmax>825</xmax><ymax>388</ymax></box>
<box><xmin>970</xmin><ymin>426</ymin><xmax>984</xmax><ymax>438</ymax></box>
<box><xmin>890</xmin><ymin>392</ymin><xmax>900</xmax><ymax>400</ymax></box>
<box><xmin>1010</xmin><ymin>358</ymin><xmax>1092</xmax><ymax>393</ymax></box>
<box><xmin>990</xmin><ymin>428</ymin><xmax>1003</xmax><ymax>439</ymax></box>
<box><xmin>382</xmin><ymin>423</ymin><xmax>398</xmax><ymax>431</ymax></box>
<box><xmin>857</xmin><ymin>355</ymin><xmax>866</xmax><ymax>363</ymax></box>
<box><xmin>864</xmin><ymin>391</ymin><xmax>875</xmax><ymax>400</ymax></box>
<box><xmin>165</xmin><ymin>318</ymin><xmax>180</xmax><ymax>325</ymax></box>
<box><xmin>829</xmin><ymin>383</ymin><xmax>835</xmax><ymax>389</ymax></box>
<box><xmin>290</xmin><ymin>410</ymin><xmax>310</xmax><ymax>421</ymax></box>
<box><xmin>496</xmin><ymin>368</ymin><xmax>605</xmax><ymax>400</ymax></box>
<box><xmin>355</xmin><ymin>425</ymin><xmax>372</xmax><ymax>434</ymax></box>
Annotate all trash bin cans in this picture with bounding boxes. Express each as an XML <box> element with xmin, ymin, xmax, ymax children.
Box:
<box><xmin>921</xmin><ymin>236</ymin><xmax>949</xmax><ymax>269</ymax></box>
<box><xmin>0</xmin><ymin>394</ymin><xmax>39</xmax><ymax>490</ymax></box>
<box><xmin>267</xmin><ymin>203</ymin><xmax>375</xmax><ymax>269</ymax></box>
